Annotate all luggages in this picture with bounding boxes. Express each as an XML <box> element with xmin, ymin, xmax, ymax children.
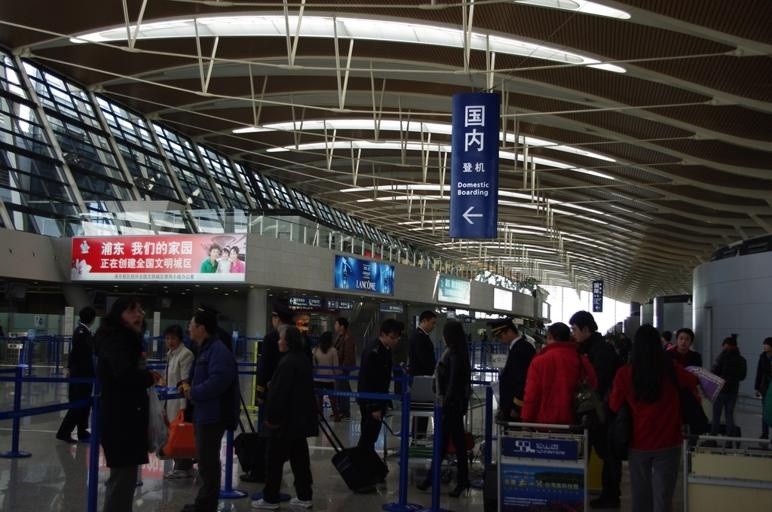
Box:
<box><xmin>314</xmin><ymin>409</ymin><xmax>392</xmax><ymax>495</ymax></box>
<box><xmin>231</xmin><ymin>391</ymin><xmax>270</xmax><ymax>482</ymax></box>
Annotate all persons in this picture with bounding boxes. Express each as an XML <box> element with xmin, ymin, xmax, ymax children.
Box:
<box><xmin>199</xmin><ymin>243</ymin><xmax>220</xmax><ymax>273</ymax></box>
<box><xmin>217</xmin><ymin>247</ymin><xmax>231</xmax><ymax>272</ymax></box>
<box><xmin>754</xmin><ymin>336</ymin><xmax>772</xmax><ymax>446</ymax></box>
<box><xmin>230</xmin><ymin>246</ymin><xmax>245</xmax><ymax>273</ymax></box>
<box><xmin>54</xmin><ymin>297</ymin><xmax>357</xmax><ymax>512</ymax></box>
<box><xmin>487</xmin><ymin>311</ymin><xmax>709</xmax><ymax>512</ymax></box>
<box><xmin>710</xmin><ymin>336</ymin><xmax>747</xmax><ymax>437</ymax></box>
<box><xmin>356</xmin><ymin>311</ymin><xmax>471</xmax><ymax>499</ymax></box>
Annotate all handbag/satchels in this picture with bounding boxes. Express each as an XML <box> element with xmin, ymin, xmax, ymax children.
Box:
<box><xmin>162</xmin><ymin>409</ymin><xmax>199</xmax><ymax>460</ymax></box>
<box><xmin>574</xmin><ymin>356</ymin><xmax>607</xmax><ymax>435</ymax></box>
<box><xmin>446</xmin><ymin>431</ymin><xmax>475</xmax><ymax>454</ymax></box>
<box><xmin>609</xmin><ymin>400</ymin><xmax>633</xmax><ymax>447</ymax></box>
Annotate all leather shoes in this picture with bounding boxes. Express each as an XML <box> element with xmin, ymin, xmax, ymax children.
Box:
<box><xmin>53</xmin><ymin>430</ymin><xmax>74</xmax><ymax>444</ymax></box>
<box><xmin>77</xmin><ymin>428</ymin><xmax>93</xmax><ymax>442</ymax></box>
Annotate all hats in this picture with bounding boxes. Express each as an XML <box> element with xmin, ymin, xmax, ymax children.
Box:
<box><xmin>268</xmin><ymin>301</ymin><xmax>298</xmax><ymax>318</ymax></box>
<box><xmin>197</xmin><ymin>302</ymin><xmax>223</xmax><ymax>319</ymax></box>
<box><xmin>486</xmin><ymin>317</ymin><xmax>516</xmax><ymax>335</ymax></box>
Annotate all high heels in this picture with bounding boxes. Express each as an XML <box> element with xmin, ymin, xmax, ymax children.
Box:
<box><xmin>449</xmin><ymin>474</ymin><xmax>472</xmax><ymax>498</ymax></box>
<box><xmin>414</xmin><ymin>467</ymin><xmax>441</xmax><ymax>491</ymax></box>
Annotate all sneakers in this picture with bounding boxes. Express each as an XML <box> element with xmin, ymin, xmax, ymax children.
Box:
<box><xmin>250</xmin><ymin>494</ymin><xmax>282</xmax><ymax>511</ymax></box>
<box><xmin>177</xmin><ymin>499</ymin><xmax>219</xmax><ymax>512</ymax></box>
<box><xmin>164</xmin><ymin>466</ymin><xmax>200</xmax><ymax>479</ymax></box>
<box><xmin>589</xmin><ymin>495</ymin><xmax>621</xmax><ymax>510</ymax></box>
<box><xmin>289</xmin><ymin>496</ymin><xmax>313</xmax><ymax>511</ymax></box>
